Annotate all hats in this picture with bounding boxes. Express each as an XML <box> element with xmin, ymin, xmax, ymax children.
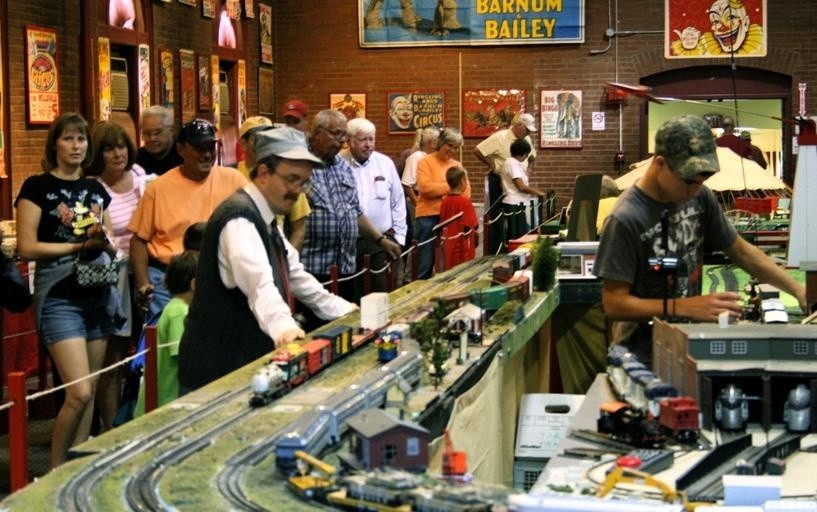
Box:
<box><xmin>741</xmin><ymin>131</ymin><xmax>750</xmax><ymax>140</ymax></box>
<box><xmin>281</xmin><ymin>100</ymin><xmax>309</xmax><ymax>119</ymax></box>
<box><xmin>181</xmin><ymin>118</ymin><xmax>219</xmax><ymax>147</ymax></box>
<box><xmin>655</xmin><ymin>114</ymin><xmax>720</xmax><ymax>179</ymax></box>
<box><xmin>240</xmin><ymin>116</ymin><xmax>272</xmax><ymax>138</ymax></box>
<box><xmin>253</xmin><ymin>128</ymin><xmax>325</xmax><ymax>169</ymax></box>
<box><xmin>518</xmin><ymin>113</ymin><xmax>537</xmax><ymax>132</ymax></box>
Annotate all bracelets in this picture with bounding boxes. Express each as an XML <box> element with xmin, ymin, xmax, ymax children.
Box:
<box><xmin>663</xmin><ymin>293</ymin><xmax>668</xmax><ymax>319</ymax></box>
<box><xmin>671</xmin><ymin>297</ymin><xmax>677</xmax><ymax>317</ymax></box>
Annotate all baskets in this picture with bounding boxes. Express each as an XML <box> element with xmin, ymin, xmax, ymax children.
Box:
<box><xmin>732</xmin><ymin>196</ymin><xmax>779</xmax><ymax>212</ymax></box>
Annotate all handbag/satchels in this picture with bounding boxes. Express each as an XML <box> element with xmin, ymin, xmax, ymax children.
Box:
<box><xmin>76</xmin><ymin>234</ymin><xmax>119</xmax><ymax>286</ymax></box>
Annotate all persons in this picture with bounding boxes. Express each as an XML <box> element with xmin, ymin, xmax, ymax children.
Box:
<box><xmin>591</xmin><ymin>113</ymin><xmax>813</xmax><ymax>372</ymax></box>
<box><xmin>715</xmin><ymin>114</ymin><xmax>744</xmax><ymax>157</ymax></box>
<box><xmin>738</xmin><ymin>130</ymin><xmax>767</xmax><ymax>171</ymax></box>
<box><xmin>666</xmin><ymin>0</ymin><xmax>768</xmax><ymax>56</ymax></box>
<box><xmin>1</xmin><ymin>97</ymin><xmax>558</xmax><ymax>477</ymax></box>
<box><xmin>593</xmin><ymin>172</ymin><xmax>622</xmax><ymax>240</ymax></box>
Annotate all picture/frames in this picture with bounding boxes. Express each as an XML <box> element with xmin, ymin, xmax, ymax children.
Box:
<box><xmin>22</xmin><ymin>23</ymin><xmax>61</xmax><ymax>127</ymax></box>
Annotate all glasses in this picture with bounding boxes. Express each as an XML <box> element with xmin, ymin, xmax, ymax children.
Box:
<box><xmin>274</xmin><ymin>169</ymin><xmax>313</xmax><ymax>193</ymax></box>
<box><xmin>322</xmin><ymin>123</ymin><xmax>347</xmax><ymax>140</ymax></box>
<box><xmin>142</xmin><ymin>129</ymin><xmax>162</xmax><ymax>138</ymax></box>
<box><xmin>444</xmin><ymin>141</ymin><xmax>458</xmax><ymax>149</ymax></box>
